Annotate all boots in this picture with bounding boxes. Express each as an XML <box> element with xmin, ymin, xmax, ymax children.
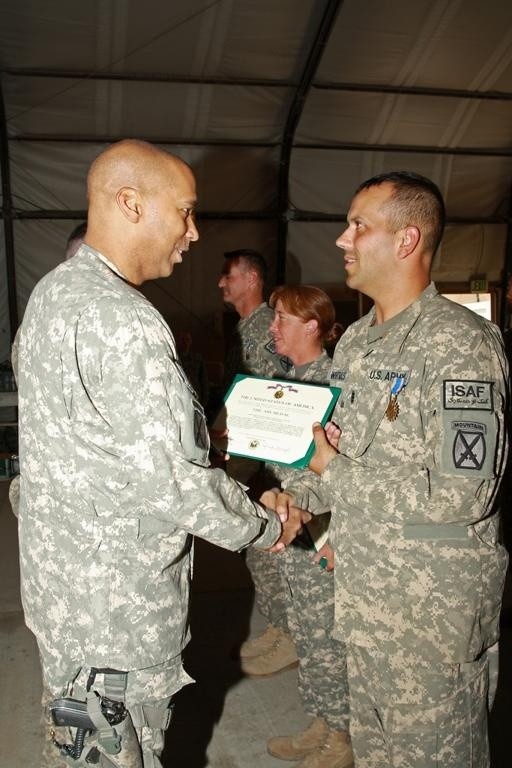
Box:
<box><xmin>236</xmin><ymin>623</ymin><xmax>300</xmax><ymax>679</ymax></box>
<box><xmin>266</xmin><ymin>715</ymin><xmax>329</xmax><ymax>761</ymax></box>
<box><xmin>297</xmin><ymin>728</ymin><xmax>355</xmax><ymax>768</ymax></box>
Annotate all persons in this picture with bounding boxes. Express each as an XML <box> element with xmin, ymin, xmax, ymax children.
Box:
<box><xmin>218</xmin><ymin>246</ymin><xmax>301</xmax><ymax>675</ymax></box>
<box><xmin>20</xmin><ymin>138</ymin><xmax>315</xmax><ymax>766</ymax></box>
<box><xmin>7</xmin><ymin>221</ymin><xmax>86</xmax><ymax>519</ymax></box>
<box><xmin>263</xmin><ymin>170</ymin><xmax>511</xmax><ymax>766</ymax></box>
<box><xmin>268</xmin><ymin>276</ymin><xmax>355</xmax><ymax>765</ymax></box>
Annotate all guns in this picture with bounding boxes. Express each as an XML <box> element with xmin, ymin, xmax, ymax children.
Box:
<box><xmin>49</xmin><ymin>698</ymin><xmax>125</xmax><ymax>730</ymax></box>
